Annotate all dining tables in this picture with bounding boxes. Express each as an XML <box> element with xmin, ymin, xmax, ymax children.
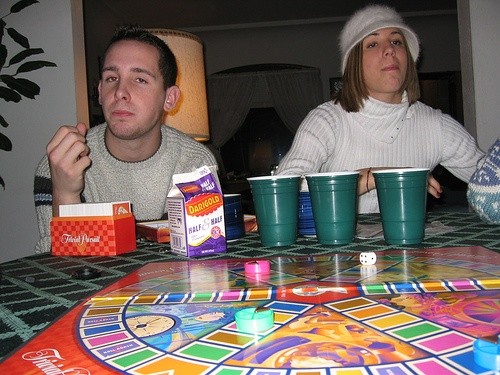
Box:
<box><xmin>0</xmin><ymin>209</ymin><xmax>500</xmax><ymax>375</ymax></box>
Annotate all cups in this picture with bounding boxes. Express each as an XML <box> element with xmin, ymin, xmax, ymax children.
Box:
<box><xmin>298</xmin><ymin>192</ymin><xmax>316</xmax><ymax>237</ymax></box>
<box><xmin>372</xmin><ymin>169</ymin><xmax>430</xmax><ymax>245</ymax></box>
<box><xmin>246</xmin><ymin>175</ymin><xmax>301</xmax><ymax>248</ymax></box>
<box><xmin>224</xmin><ymin>193</ymin><xmax>245</xmax><ymax>239</ymax></box>
<box><xmin>305</xmin><ymin>172</ymin><xmax>361</xmax><ymax>245</ymax></box>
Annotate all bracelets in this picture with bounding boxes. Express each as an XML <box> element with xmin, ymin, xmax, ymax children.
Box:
<box><xmin>366</xmin><ymin>166</ymin><xmax>371</xmax><ymax>193</ymax></box>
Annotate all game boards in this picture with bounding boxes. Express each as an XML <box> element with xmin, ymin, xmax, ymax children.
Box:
<box><xmin>0</xmin><ymin>246</ymin><xmax>500</xmax><ymax>375</ymax></box>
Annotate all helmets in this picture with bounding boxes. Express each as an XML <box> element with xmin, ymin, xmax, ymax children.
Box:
<box><xmin>338</xmin><ymin>7</ymin><xmax>421</xmax><ymax>77</ymax></box>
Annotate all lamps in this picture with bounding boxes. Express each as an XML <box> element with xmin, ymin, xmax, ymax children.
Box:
<box><xmin>142</xmin><ymin>29</ymin><xmax>210</xmax><ymax>142</ymax></box>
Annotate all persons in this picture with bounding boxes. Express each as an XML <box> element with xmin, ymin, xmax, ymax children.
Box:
<box><xmin>270</xmin><ymin>4</ymin><xmax>484</xmax><ymax>215</ymax></box>
<box><xmin>33</xmin><ymin>28</ymin><xmax>220</xmax><ymax>253</ymax></box>
